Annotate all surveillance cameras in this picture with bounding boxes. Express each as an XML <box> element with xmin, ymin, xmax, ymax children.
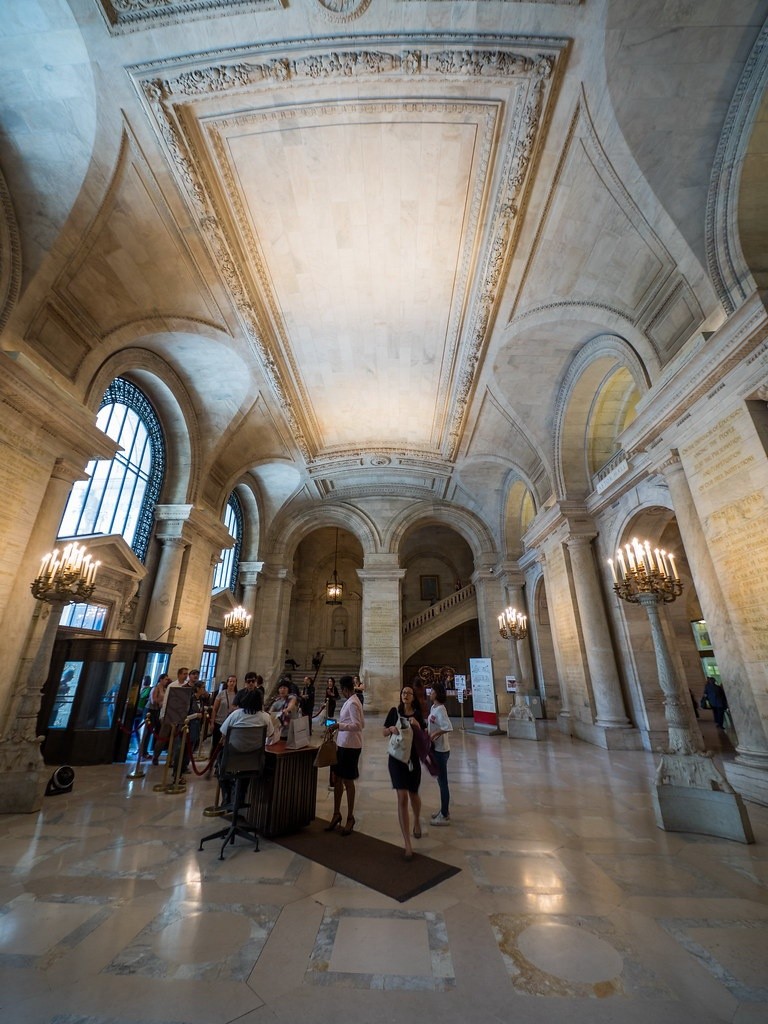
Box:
<box><xmin>489</xmin><ymin>568</ymin><xmax>493</xmax><ymax>573</ymax></box>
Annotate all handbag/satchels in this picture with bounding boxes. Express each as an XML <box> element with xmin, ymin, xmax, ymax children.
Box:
<box><xmin>333</xmin><ymin>695</ymin><xmax>341</xmax><ymax>700</ymax></box>
<box><xmin>385</xmin><ymin>706</ymin><xmax>413</xmax><ymax>763</ymax></box>
<box><xmin>701</xmin><ymin>695</ymin><xmax>711</xmax><ymax>709</ymax></box>
<box><xmin>314</xmin><ymin>729</ymin><xmax>338</xmax><ymax>768</ymax></box>
<box><xmin>285</xmin><ymin>715</ymin><xmax>310</xmax><ymax>750</ymax></box>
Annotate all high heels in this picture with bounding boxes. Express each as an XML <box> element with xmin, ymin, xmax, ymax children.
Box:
<box><xmin>341</xmin><ymin>815</ymin><xmax>356</xmax><ymax>836</ymax></box>
<box><xmin>323</xmin><ymin>815</ymin><xmax>342</xmax><ymax>832</ymax></box>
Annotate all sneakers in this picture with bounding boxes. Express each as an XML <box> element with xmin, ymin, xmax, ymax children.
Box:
<box><xmin>433</xmin><ymin>808</ymin><xmax>450</xmax><ymax>817</ymax></box>
<box><xmin>430</xmin><ymin>812</ymin><xmax>449</xmax><ymax>827</ymax></box>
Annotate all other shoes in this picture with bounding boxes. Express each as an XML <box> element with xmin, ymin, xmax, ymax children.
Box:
<box><xmin>412</xmin><ymin>825</ymin><xmax>421</xmax><ymax>837</ymax></box>
<box><xmin>154</xmin><ymin>757</ymin><xmax>158</xmax><ymax>765</ymax></box>
<box><xmin>400</xmin><ymin>849</ymin><xmax>413</xmax><ymax>862</ymax></box>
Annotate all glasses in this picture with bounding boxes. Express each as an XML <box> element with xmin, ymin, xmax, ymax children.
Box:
<box><xmin>244</xmin><ymin>679</ymin><xmax>256</xmax><ymax>684</ymax></box>
<box><xmin>402</xmin><ymin>691</ymin><xmax>413</xmax><ymax>696</ymax></box>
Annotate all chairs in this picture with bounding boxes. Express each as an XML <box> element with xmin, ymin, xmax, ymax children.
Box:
<box><xmin>197</xmin><ymin>724</ymin><xmax>268</xmax><ymax>859</ymax></box>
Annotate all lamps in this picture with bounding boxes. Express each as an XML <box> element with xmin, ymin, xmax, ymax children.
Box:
<box><xmin>497</xmin><ymin>606</ymin><xmax>527</xmax><ymax>642</ymax></box>
<box><xmin>605</xmin><ymin>535</ymin><xmax>683</xmax><ymax>604</ymax></box>
<box><xmin>30</xmin><ymin>539</ymin><xmax>102</xmax><ymax>607</ymax></box>
<box><xmin>222</xmin><ymin>605</ymin><xmax>252</xmax><ymax>638</ymax></box>
<box><xmin>326</xmin><ymin>527</ymin><xmax>342</xmax><ymax>606</ymax></box>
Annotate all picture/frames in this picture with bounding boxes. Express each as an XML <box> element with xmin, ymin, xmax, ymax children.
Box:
<box><xmin>420</xmin><ymin>575</ymin><xmax>440</xmax><ymax>601</ymax></box>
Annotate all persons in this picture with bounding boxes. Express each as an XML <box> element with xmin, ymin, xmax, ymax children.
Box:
<box><xmin>427</xmin><ymin>683</ymin><xmax>454</xmax><ymax>826</ymax></box>
<box><xmin>214</xmin><ymin>689</ymin><xmax>275</xmax><ymax>811</ymax></box>
<box><xmin>324</xmin><ymin>676</ymin><xmax>341</xmax><ymax>719</ymax></box>
<box><xmin>268</xmin><ymin>673</ymin><xmax>315</xmax><ymax>741</ymax></box>
<box><xmin>132</xmin><ymin>667</ymin><xmax>238</xmax><ymax>786</ymax></box>
<box><xmin>229</xmin><ymin>672</ymin><xmax>265</xmax><ymax>712</ymax></box>
<box><xmin>107</xmin><ymin>680</ymin><xmax>121</xmax><ymax>727</ymax></box>
<box><xmin>255</xmin><ymin>674</ymin><xmax>265</xmax><ymax>696</ymax></box>
<box><xmin>383</xmin><ymin>685</ymin><xmax>426</xmax><ymax>861</ymax></box>
<box><xmin>284</xmin><ymin>649</ymin><xmax>301</xmax><ymax>670</ymax></box>
<box><xmin>323</xmin><ymin>676</ymin><xmax>365</xmax><ymax>836</ymax></box>
<box><xmin>353</xmin><ymin>676</ymin><xmax>365</xmax><ymax>707</ymax></box>
<box><xmin>48</xmin><ymin>670</ymin><xmax>74</xmax><ymax>726</ymax></box>
<box><xmin>700</xmin><ymin>676</ymin><xmax>729</xmax><ymax>729</ymax></box>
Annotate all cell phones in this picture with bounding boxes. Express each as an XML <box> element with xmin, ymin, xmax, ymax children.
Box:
<box><xmin>325</xmin><ymin>718</ymin><xmax>337</xmax><ymax>726</ymax></box>
